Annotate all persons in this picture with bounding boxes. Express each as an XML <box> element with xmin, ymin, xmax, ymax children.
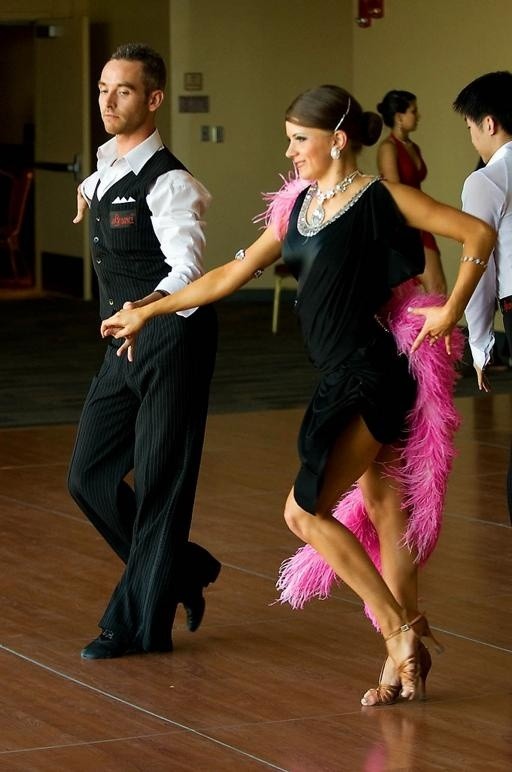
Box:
<box><xmin>454</xmin><ymin>68</ymin><xmax>512</xmax><ymax>396</ymax></box>
<box><xmin>374</xmin><ymin>88</ymin><xmax>449</xmax><ymax>297</ymax></box>
<box><xmin>63</xmin><ymin>42</ymin><xmax>226</xmax><ymax>662</ymax></box>
<box><xmin>96</xmin><ymin>83</ymin><xmax>500</xmax><ymax>710</ymax></box>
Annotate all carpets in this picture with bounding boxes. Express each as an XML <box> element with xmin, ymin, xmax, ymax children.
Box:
<box><xmin>0</xmin><ymin>298</ymin><xmax>510</xmax><ymax>434</ymax></box>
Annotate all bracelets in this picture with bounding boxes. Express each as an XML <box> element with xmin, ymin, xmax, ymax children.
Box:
<box><xmin>460</xmin><ymin>255</ymin><xmax>489</xmax><ymax>272</ymax></box>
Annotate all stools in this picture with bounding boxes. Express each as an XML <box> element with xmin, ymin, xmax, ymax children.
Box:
<box><xmin>266</xmin><ymin>258</ymin><xmax>304</xmax><ymax>337</ymax></box>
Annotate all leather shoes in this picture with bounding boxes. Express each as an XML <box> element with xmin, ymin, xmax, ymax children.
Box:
<box><xmin>180</xmin><ymin>542</ymin><xmax>222</xmax><ymax>633</ymax></box>
<box><xmin>80</xmin><ymin>628</ymin><xmax>141</xmax><ymax>660</ymax></box>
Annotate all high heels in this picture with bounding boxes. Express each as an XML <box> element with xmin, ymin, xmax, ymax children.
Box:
<box><xmin>383</xmin><ymin>608</ymin><xmax>447</xmax><ymax>702</ymax></box>
<box><xmin>360</xmin><ymin>643</ymin><xmax>433</xmax><ymax>707</ymax></box>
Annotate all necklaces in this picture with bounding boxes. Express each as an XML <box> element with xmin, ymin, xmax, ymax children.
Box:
<box><xmin>311</xmin><ymin>169</ymin><xmax>360</xmax><ymax>228</ymax></box>
